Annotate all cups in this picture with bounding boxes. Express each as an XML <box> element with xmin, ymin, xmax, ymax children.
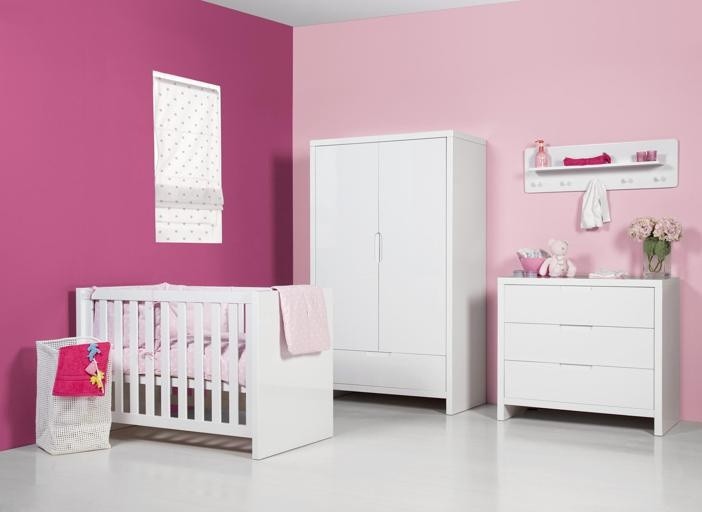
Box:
<box><xmin>646</xmin><ymin>150</ymin><xmax>657</xmax><ymax>161</ymax></box>
<box><xmin>637</xmin><ymin>151</ymin><xmax>646</xmax><ymax>162</ymax></box>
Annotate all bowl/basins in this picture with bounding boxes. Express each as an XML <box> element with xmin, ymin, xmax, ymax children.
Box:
<box><xmin>519</xmin><ymin>257</ymin><xmax>550</xmax><ymax>274</ymax></box>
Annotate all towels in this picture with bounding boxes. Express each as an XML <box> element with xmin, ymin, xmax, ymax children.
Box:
<box><xmin>579</xmin><ymin>178</ymin><xmax>611</xmax><ymax>229</ymax></box>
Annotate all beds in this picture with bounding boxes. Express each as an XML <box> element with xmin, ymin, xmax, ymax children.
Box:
<box><xmin>73</xmin><ymin>286</ymin><xmax>334</xmax><ymax>459</ymax></box>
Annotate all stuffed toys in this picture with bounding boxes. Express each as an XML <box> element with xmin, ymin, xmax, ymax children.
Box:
<box><xmin>538</xmin><ymin>239</ymin><xmax>577</xmax><ymax>277</ymax></box>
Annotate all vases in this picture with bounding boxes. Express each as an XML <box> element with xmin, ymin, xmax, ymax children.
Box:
<box><xmin>643</xmin><ymin>245</ymin><xmax>671</xmax><ymax>278</ymax></box>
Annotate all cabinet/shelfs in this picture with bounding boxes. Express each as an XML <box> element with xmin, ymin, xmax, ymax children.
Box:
<box><xmin>308</xmin><ymin>130</ymin><xmax>488</xmax><ymax>415</ymax></box>
<box><xmin>495</xmin><ymin>277</ymin><xmax>681</xmax><ymax>437</ymax></box>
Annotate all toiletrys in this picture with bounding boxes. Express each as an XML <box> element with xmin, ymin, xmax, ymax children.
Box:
<box><xmin>534</xmin><ymin>139</ymin><xmax>548</xmax><ymax>168</ymax></box>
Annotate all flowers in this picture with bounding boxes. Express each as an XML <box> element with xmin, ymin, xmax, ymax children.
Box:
<box><xmin>629</xmin><ymin>218</ymin><xmax>683</xmax><ymax>272</ymax></box>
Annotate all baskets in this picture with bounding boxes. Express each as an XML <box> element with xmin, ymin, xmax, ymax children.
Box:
<box><xmin>519</xmin><ymin>256</ymin><xmax>546</xmax><ymax>271</ymax></box>
